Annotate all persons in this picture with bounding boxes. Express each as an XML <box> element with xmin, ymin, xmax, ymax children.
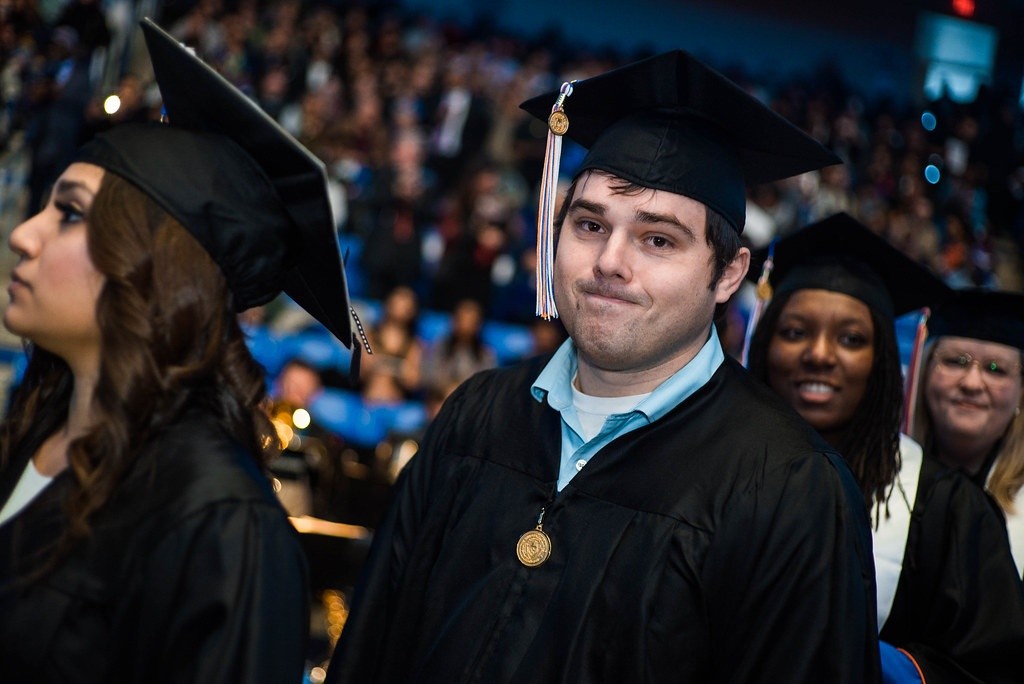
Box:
<box><xmin>903</xmin><ymin>283</ymin><xmax>1024</xmax><ymax>578</ymax></box>
<box><xmin>0</xmin><ymin>0</ymin><xmax>567</xmax><ymax>651</ymax></box>
<box><xmin>726</xmin><ymin>63</ymin><xmax>1024</xmax><ymax>385</ymax></box>
<box><xmin>735</xmin><ymin>211</ymin><xmax>1024</xmax><ymax>684</ymax></box>
<box><xmin>325</xmin><ymin>51</ymin><xmax>883</xmax><ymax>684</ymax></box>
<box><xmin>0</xmin><ymin>17</ymin><xmax>371</xmax><ymax>684</ymax></box>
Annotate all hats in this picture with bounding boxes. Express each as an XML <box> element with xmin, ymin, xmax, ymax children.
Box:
<box><xmin>68</xmin><ymin>14</ymin><xmax>354</xmax><ymax>350</ymax></box>
<box><xmin>906</xmin><ymin>290</ymin><xmax>1024</xmax><ymax>439</ymax></box>
<box><xmin>519</xmin><ymin>49</ymin><xmax>845</xmax><ymax>324</ymax></box>
<box><xmin>739</xmin><ymin>213</ymin><xmax>957</xmax><ymax>439</ymax></box>
